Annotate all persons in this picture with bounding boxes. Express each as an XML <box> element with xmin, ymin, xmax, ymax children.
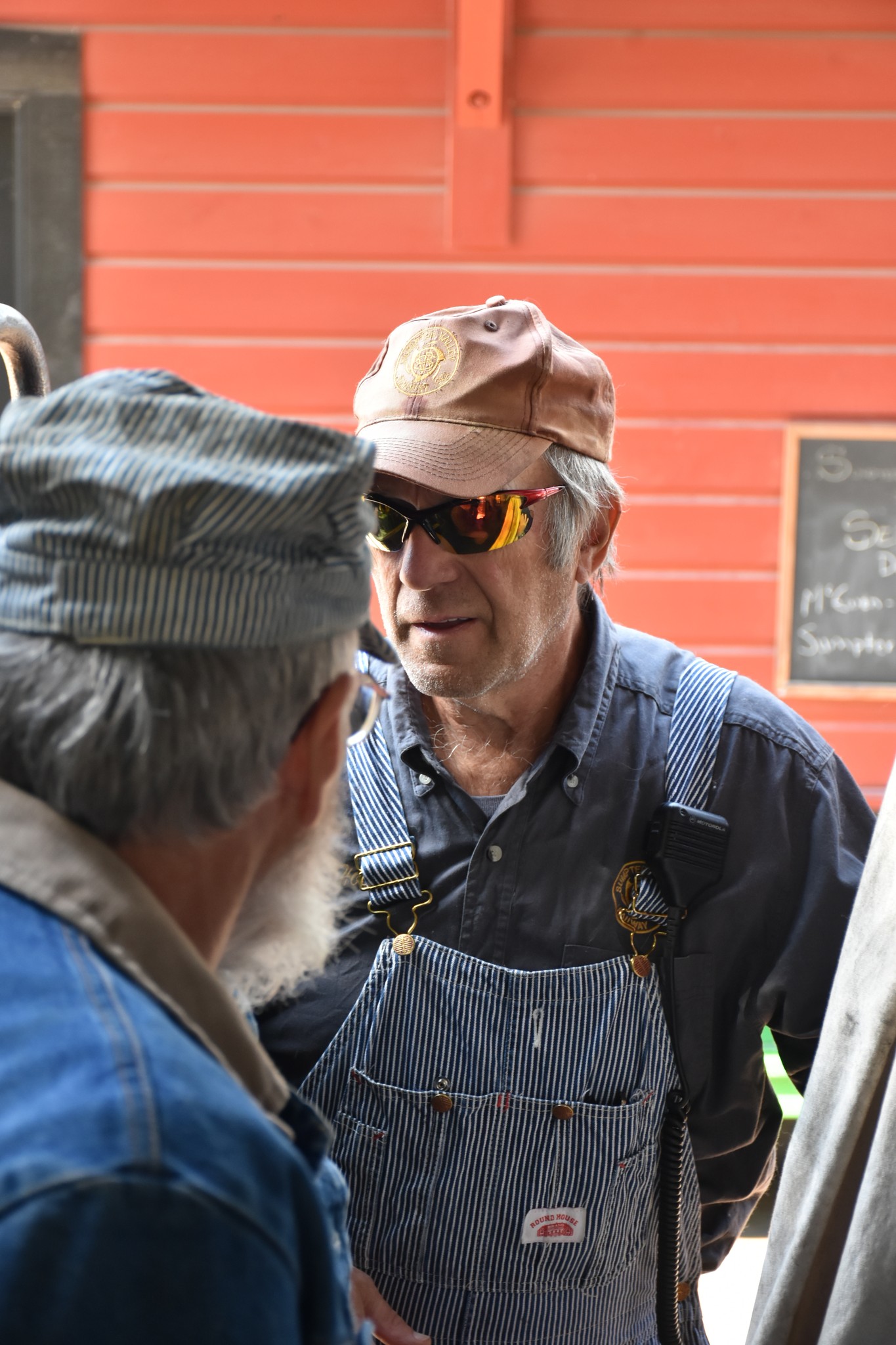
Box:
<box><xmin>203</xmin><ymin>298</ymin><xmax>880</xmax><ymax>1345</ymax></box>
<box><xmin>0</xmin><ymin>367</ymin><xmax>379</xmax><ymax>1345</ymax></box>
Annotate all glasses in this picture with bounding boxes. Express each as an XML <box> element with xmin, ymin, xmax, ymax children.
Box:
<box><xmin>365</xmin><ymin>487</ymin><xmax>568</xmax><ymax>552</ymax></box>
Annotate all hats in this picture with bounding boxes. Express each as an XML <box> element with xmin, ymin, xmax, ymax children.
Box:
<box><xmin>0</xmin><ymin>369</ymin><xmax>403</xmax><ymax>669</ymax></box>
<box><xmin>350</xmin><ymin>296</ymin><xmax>614</xmax><ymax>497</ymax></box>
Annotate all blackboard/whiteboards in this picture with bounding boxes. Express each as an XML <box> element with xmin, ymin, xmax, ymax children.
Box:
<box><xmin>776</xmin><ymin>422</ymin><xmax>896</xmax><ymax>698</ymax></box>
